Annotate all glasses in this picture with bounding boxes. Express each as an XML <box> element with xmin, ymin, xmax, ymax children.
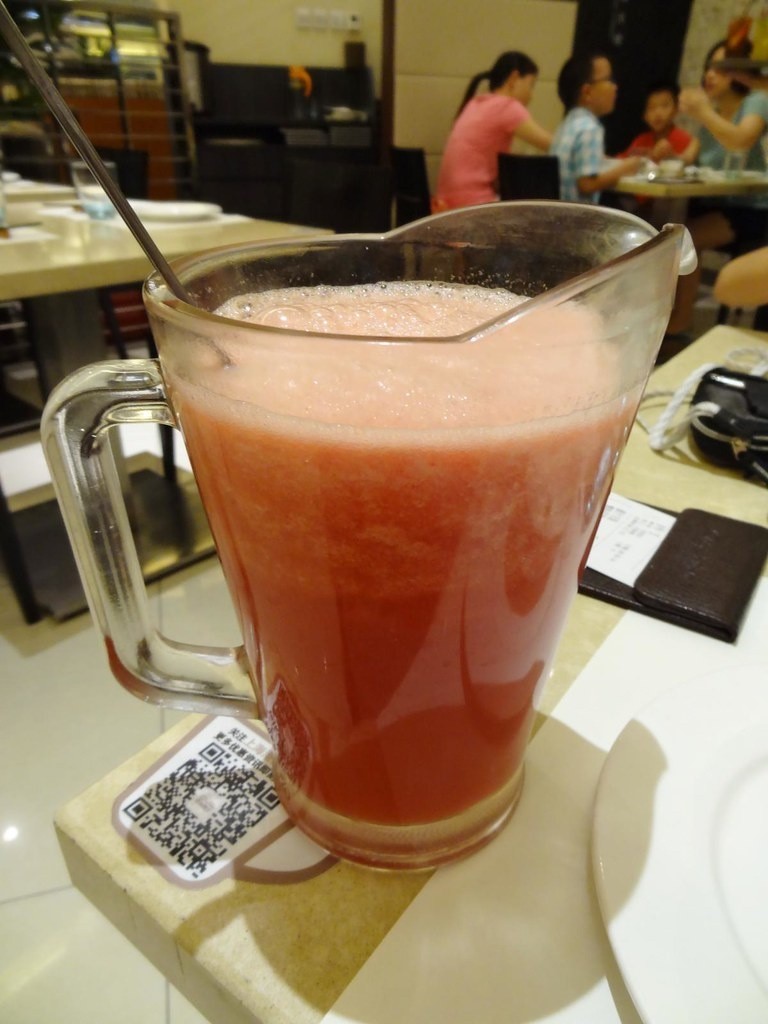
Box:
<box><xmin>588</xmin><ymin>76</ymin><xmax>617</xmax><ymax>84</ymax></box>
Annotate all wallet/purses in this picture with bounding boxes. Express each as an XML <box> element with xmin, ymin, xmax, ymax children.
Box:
<box><xmin>651</xmin><ymin>349</ymin><xmax>768</xmax><ymax>483</ymax></box>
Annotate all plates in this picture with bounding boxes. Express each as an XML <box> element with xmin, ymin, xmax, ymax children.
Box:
<box><xmin>644</xmin><ymin>175</ymin><xmax>704</xmax><ymax>184</ymax></box>
<box><xmin>592</xmin><ymin>651</ymin><xmax>767</xmax><ymax>1024</ymax></box>
<box><xmin>131</xmin><ymin>201</ymin><xmax>222</xmax><ymax>220</ymax></box>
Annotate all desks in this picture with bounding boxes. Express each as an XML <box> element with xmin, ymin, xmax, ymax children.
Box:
<box><xmin>606</xmin><ymin>157</ymin><xmax>767</xmax><ymax>231</ymax></box>
<box><xmin>0</xmin><ymin>170</ymin><xmax>337</xmax><ymax>623</ymax></box>
<box><xmin>0</xmin><ymin>324</ymin><xmax>765</xmax><ymax>1023</ymax></box>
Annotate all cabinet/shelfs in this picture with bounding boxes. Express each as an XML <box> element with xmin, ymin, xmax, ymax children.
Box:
<box><xmin>198</xmin><ymin>116</ymin><xmax>393</xmax><ymax>234</ymax></box>
<box><xmin>0</xmin><ymin>0</ymin><xmax>199</xmax><ymax>201</ymax></box>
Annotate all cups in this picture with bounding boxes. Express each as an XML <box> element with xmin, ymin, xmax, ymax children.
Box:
<box><xmin>724</xmin><ymin>153</ymin><xmax>745</xmax><ymax>177</ymax></box>
<box><xmin>71</xmin><ymin>160</ymin><xmax>120</xmax><ymax>222</ymax></box>
<box><xmin>41</xmin><ymin>202</ymin><xmax>686</xmax><ymax>872</ymax></box>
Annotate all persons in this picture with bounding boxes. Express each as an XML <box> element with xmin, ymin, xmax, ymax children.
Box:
<box><xmin>556</xmin><ymin>50</ymin><xmax>649</xmax><ymax>213</ymax></box>
<box><xmin>615</xmin><ymin>34</ymin><xmax>768</xmax><ymax>363</ymax></box>
<box><xmin>427</xmin><ymin>46</ymin><xmax>553</xmax><ymax>212</ymax></box>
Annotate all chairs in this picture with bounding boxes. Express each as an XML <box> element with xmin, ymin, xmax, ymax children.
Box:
<box><xmin>493</xmin><ymin>153</ymin><xmax>561</xmax><ymax>201</ymax></box>
<box><xmin>382</xmin><ymin>147</ymin><xmax>431</xmax><ymax>230</ymax></box>
<box><xmin>87</xmin><ymin>147</ymin><xmax>151</xmax><ymax>198</ymax></box>
